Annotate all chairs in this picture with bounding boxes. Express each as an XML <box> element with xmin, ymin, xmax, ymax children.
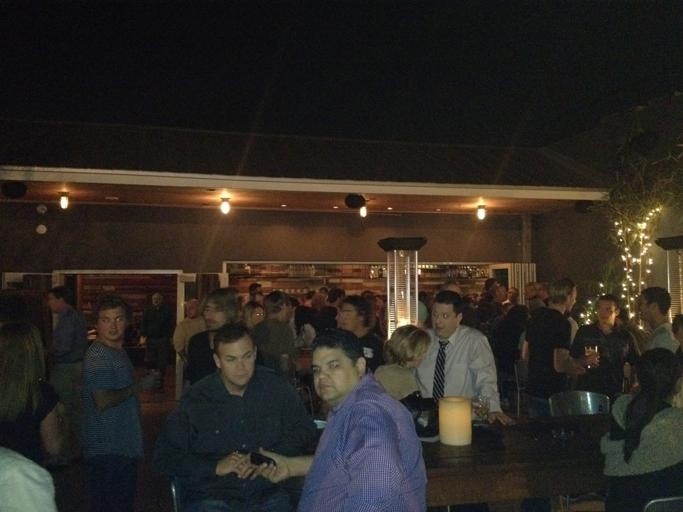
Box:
<box><xmin>512</xmin><ymin>359</ymin><xmax>526</xmax><ymax>419</ymax></box>
<box><xmin>278</xmin><ymin>355</ymin><xmax>314</xmax><ymax>417</ymax></box>
<box><xmin>643</xmin><ymin>497</ymin><xmax>682</xmax><ymax>512</ymax></box>
<box><xmin>548</xmin><ymin>390</ymin><xmax>609</xmax><ymax>511</ymax></box>
<box><xmin>170</xmin><ymin>482</ymin><xmax>182</xmax><ymax>512</ymax></box>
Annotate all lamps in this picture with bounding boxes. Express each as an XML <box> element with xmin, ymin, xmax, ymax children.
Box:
<box><xmin>359</xmin><ymin>200</ymin><xmax>367</xmax><ymax>218</ymax></box>
<box><xmin>477</xmin><ymin>205</ymin><xmax>486</xmax><ymax>220</ymax></box>
<box><xmin>220</xmin><ymin>198</ymin><xmax>231</xmax><ymax>214</ymax></box>
<box><xmin>59</xmin><ymin>191</ymin><xmax>69</xmax><ymax>210</ymax></box>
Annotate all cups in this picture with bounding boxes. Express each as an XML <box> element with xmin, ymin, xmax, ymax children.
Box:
<box><xmin>585</xmin><ymin>345</ymin><xmax>600</xmax><ymax>368</ymax></box>
<box><xmin>149</xmin><ymin>369</ymin><xmax>163</xmax><ymax>391</ymax></box>
<box><xmin>472</xmin><ymin>396</ymin><xmax>490</xmax><ymax>426</ymax></box>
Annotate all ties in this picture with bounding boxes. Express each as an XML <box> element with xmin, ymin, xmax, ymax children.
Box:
<box><xmin>432</xmin><ymin>340</ymin><xmax>449</xmax><ymax>403</ymax></box>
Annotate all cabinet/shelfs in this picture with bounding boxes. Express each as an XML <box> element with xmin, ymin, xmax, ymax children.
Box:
<box><xmin>222</xmin><ymin>260</ymin><xmax>494</xmax><ymax>304</ymax></box>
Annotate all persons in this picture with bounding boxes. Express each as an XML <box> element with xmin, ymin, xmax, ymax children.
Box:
<box><xmin>621</xmin><ymin>287</ymin><xmax>677</xmax><ymax>355</ymax></box>
<box><xmin>0</xmin><ymin>443</ymin><xmax>56</xmax><ymax>512</ymax></box>
<box><xmin>76</xmin><ymin>295</ymin><xmax>162</xmax><ymax>512</ymax></box>
<box><xmin>256</xmin><ymin>290</ymin><xmax>303</xmax><ymax>374</ymax></box>
<box><xmin>183</xmin><ymin>288</ymin><xmax>266</xmax><ymax>397</ymax></box>
<box><xmin>249</xmin><ymin>283</ymin><xmax>263</xmax><ymax>301</ymax></box>
<box><xmin>598</xmin><ymin>346</ymin><xmax>682</xmax><ymax>512</ymax></box>
<box><xmin>369</xmin><ymin>327</ymin><xmax>438</xmax><ymax>418</ymax></box>
<box><xmin>139</xmin><ymin>290</ymin><xmax>174</xmax><ymax>393</ymax></box>
<box><xmin>45</xmin><ymin>286</ymin><xmax>87</xmax><ymax>437</ymax></box>
<box><xmin>251</xmin><ymin>325</ymin><xmax>429</xmax><ymax>512</ymax></box>
<box><xmin>672</xmin><ymin>313</ymin><xmax>682</xmax><ymax>348</ymax></box>
<box><xmin>173</xmin><ymin>295</ymin><xmax>207</xmax><ymax>401</ymax></box>
<box><xmin>151</xmin><ymin>321</ymin><xmax>321</xmax><ymax>511</ymax></box>
<box><xmin>337</xmin><ymin>295</ymin><xmax>387</xmax><ymax>373</ymax></box>
<box><xmin>242</xmin><ymin>301</ymin><xmax>263</xmax><ymax>332</ymax></box>
<box><xmin>521</xmin><ymin>279</ymin><xmax>598</xmax><ymax>418</ymax></box>
<box><xmin>0</xmin><ymin>321</ymin><xmax>65</xmax><ymax>464</ymax></box>
<box><xmin>415</xmin><ymin>290</ymin><xmax>518</xmax><ymax>427</ymax></box>
<box><xmin>568</xmin><ymin>292</ymin><xmax>643</xmax><ymax>429</ymax></box>
<box><xmin>297</xmin><ymin>276</ymin><xmax>551</xmax><ymax>345</ymax></box>
